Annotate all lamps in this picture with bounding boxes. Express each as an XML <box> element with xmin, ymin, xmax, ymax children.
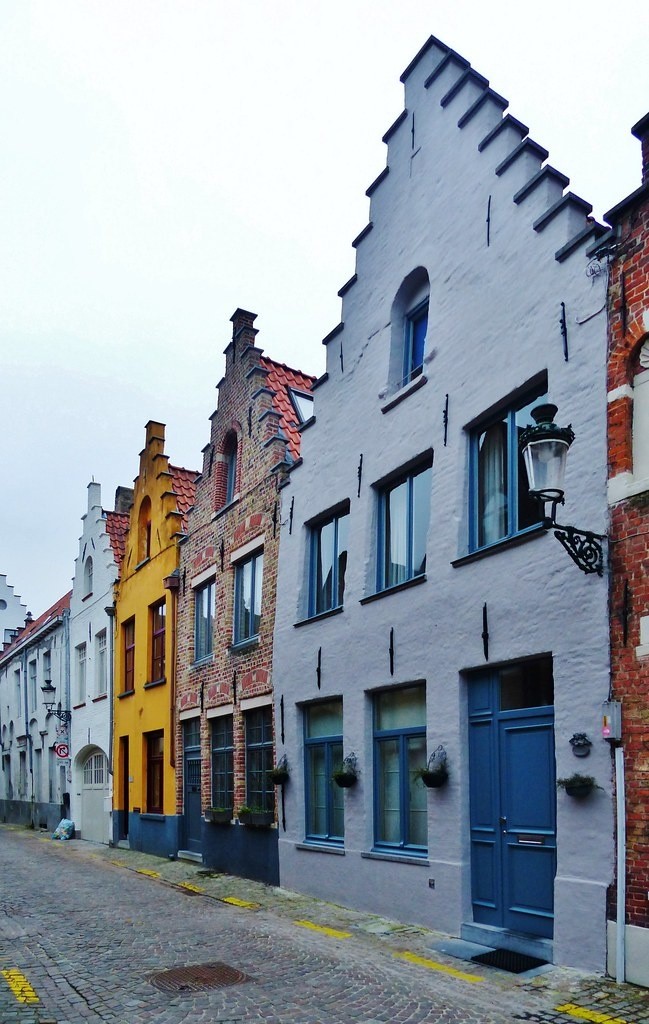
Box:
<box><xmin>517</xmin><ymin>402</ymin><xmax>606</xmax><ymax>576</ymax></box>
<box><xmin>41</xmin><ymin>679</ymin><xmax>71</xmax><ymax>723</ymax></box>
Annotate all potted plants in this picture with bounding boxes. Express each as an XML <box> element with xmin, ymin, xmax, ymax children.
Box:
<box><xmin>268</xmin><ymin>765</ymin><xmax>289</xmax><ymax>785</ymax></box>
<box><xmin>204</xmin><ymin>807</ymin><xmax>234</xmax><ymax>822</ymax></box>
<box><xmin>333</xmin><ymin>757</ymin><xmax>357</xmax><ymax>788</ymax></box>
<box><xmin>236</xmin><ymin>805</ymin><xmax>274</xmax><ymax>825</ymax></box>
<box><xmin>412</xmin><ymin>766</ymin><xmax>451</xmax><ymax>788</ymax></box>
<box><xmin>556</xmin><ymin>775</ymin><xmax>606</xmax><ymax>798</ymax></box>
